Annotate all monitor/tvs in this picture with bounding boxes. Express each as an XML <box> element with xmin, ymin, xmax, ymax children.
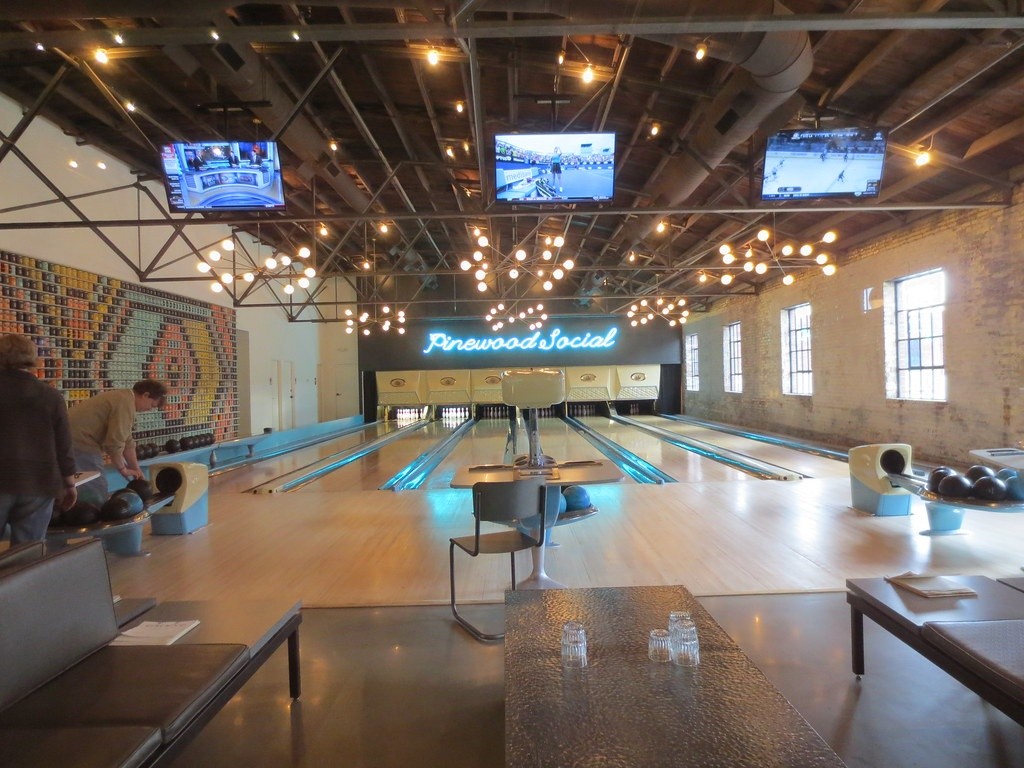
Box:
<box><xmin>760</xmin><ymin>124</ymin><xmax>890</xmax><ymax>198</ymax></box>
<box><xmin>491</xmin><ymin>130</ymin><xmax>617</xmax><ymax>206</ymax></box>
<box><xmin>156</xmin><ymin>138</ymin><xmax>288</xmax><ymax>213</ymax></box>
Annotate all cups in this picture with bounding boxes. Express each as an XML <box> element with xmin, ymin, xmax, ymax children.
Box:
<box><xmin>560</xmin><ymin>623</ymin><xmax>588</xmax><ymax>669</ymax></box>
<box><xmin>648</xmin><ymin>609</ymin><xmax>700</xmax><ymax>667</ymax></box>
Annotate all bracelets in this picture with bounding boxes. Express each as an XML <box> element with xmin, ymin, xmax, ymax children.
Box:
<box><xmin>64</xmin><ymin>482</ymin><xmax>75</xmax><ymax>488</ymax></box>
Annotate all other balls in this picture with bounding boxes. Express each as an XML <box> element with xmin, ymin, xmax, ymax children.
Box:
<box><xmin>966</xmin><ymin>465</ymin><xmax>995</xmax><ymax>482</ymax></box>
<box><xmin>938</xmin><ymin>474</ymin><xmax>971</xmax><ymax>500</ymax></box>
<box><xmin>927</xmin><ymin>466</ymin><xmax>957</xmax><ymax>492</ymax></box>
<box><xmin>68</xmin><ymin>478</ymin><xmax>152</xmax><ymax>523</ymax></box>
<box><xmin>558</xmin><ymin>493</ymin><xmax>567</xmax><ymax>514</ymax></box>
<box><xmin>996</xmin><ymin>468</ymin><xmax>1017</xmax><ymax>481</ymax></box>
<box><xmin>135</xmin><ymin>433</ymin><xmax>215</xmax><ymax>459</ymax></box>
<box><xmin>1004</xmin><ymin>476</ymin><xmax>1024</xmax><ymax>500</ymax></box>
<box><xmin>563</xmin><ymin>486</ymin><xmax>591</xmax><ymax>510</ymax></box>
<box><xmin>972</xmin><ymin>476</ymin><xmax>1007</xmax><ymax>503</ymax></box>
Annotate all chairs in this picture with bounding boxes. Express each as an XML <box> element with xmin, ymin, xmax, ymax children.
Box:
<box><xmin>449</xmin><ymin>470</ymin><xmax>547</xmax><ymax>643</ymax></box>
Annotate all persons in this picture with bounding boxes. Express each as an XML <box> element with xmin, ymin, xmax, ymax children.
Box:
<box><xmin>0</xmin><ymin>334</ymin><xmax>77</xmax><ymax>549</ymax></box>
<box><xmin>188</xmin><ymin>148</ymin><xmax>263</xmax><ymax>171</ymax></box>
<box><xmin>550</xmin><ymin>146</ymin><xmax>564</xmax><ymax>192</ymax></box>
<box><xmin>67</xmin><ymin>379</ymin><xmax>167</xmax><ymax>513</ymax></box>
<box><xmin>764</xmin><ymin>134</ymin><xmax>883</xmax><ymax>184</ymax></box>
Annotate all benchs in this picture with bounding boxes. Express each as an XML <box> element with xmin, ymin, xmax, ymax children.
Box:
<box><xmin>0</xmin><ymin>539</ymin><xmax>303</xmax><ymax>768</ymax></box>
<box><xmin>843</xmin><ymin>576</ymin><xmax>1024</xmax><ymax>729</ymax></box>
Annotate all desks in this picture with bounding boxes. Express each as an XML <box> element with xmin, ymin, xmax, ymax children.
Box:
<box><xmin>504</xmin><ymin>587</ymin><xmax>850</xmax><ymax>768</ymax></box>
